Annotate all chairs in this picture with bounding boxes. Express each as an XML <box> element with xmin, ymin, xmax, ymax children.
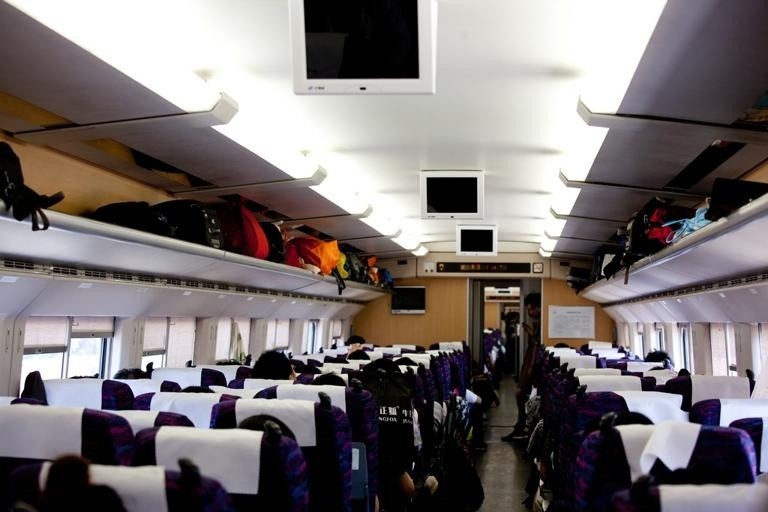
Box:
<box><xmin>589</xmin><ymin>347</ymin><xmax>626</xmax><ymax>356</ymax></box>
<box><xmin>146</xmin><ymin>362</ymin><xmax>226</xmax><ymax>389</ymax></box>
<box><xmin>209</xmin><ymin>384</ymin><xmax>262</xmax><ymax>401</ymax></box>
<box><xmin>606</xmin><ymin>356</ymin><xmax>639</xmax><ymax>367</ymax></box>
<box><xmin>318</xmin><ymin>345</ymin><xmax>350</xmax><ymax>357</ymax></box>
<box><xmin>228</xmin><ymin>378</ymin><xmax>298</xmax><ymax>392</ymax></box>
<box><xmin>689</xmin><ymin>398</ymin><xmax>766</xmax><ymax>428</ymax></box>
<box><xmin>616</xmin><ymin>358</ymin><xmax>671</xmax><ymax>371</ymax></box>
<box><xmin>358</xmin><ymin>342</ymin><xmax>374</xmax><ymax>349</ymax></box>
<box><xmin>137</xmin><ymin>420</ymin><xmax>310</xmax><ymax>512</ymax></box>
<box><xmin>418</xmin><ymin>351</ymin><xmax>454</xmax><ymax>394</ymax></box>
<box><xmin>632</xmin><ymin>369</ymin><xmax>682</xmax><ymax>387</ymax></box>
<box><xmin>561</xmin><ymin>362</ymin><xmax>617</xmax><ymax>421</ymax></box>
<box><xmin>420</xmin><ymin>349</ymin><xmax>466</xmax><ymax>395</ymax></box>
<box><xmin>666</xmin><ymin>368</ymin><xmax>756</xmax><ymax>410</ymax></box>
<box><xmin>254</xmin><ymin>376</ymin><xmax>382</xmax><ymax>512</ymax></box>
<box><xmin>296</xmin><ymin>370</ymin><xmax>387</xmax><ymax>386</ymax></box>
<box><xmin>133</xmin><ymin>390</ymin><xmax>243</xmax><ymax>427</ymax></box>
<box><xmin>104</xmin><ymin>378</ymin><xmax>180</xmax><ymax>398</ymax></box>
<box><xmin>537</xmin><ymin>343</ymin><xmax>575</xmax><ymax>392</ymax></box>
<box><xmin>574</xmin><ymin>410</ymin><xmax>759</xmax><ymax>512</ymax></box>
<box><xmin>553</xmin><ymin>357</ymin><xmax>604</xmax><ymax>406</ymax></box>
<box><xmin>729</xmin><ymin>418</ymin><xmax>766</xmax><ymax>472</ymax></box>
<box><xmin>595</xmin><ymin>351</ymin><xmax>634</xmax><ymax>362</ymax></box>
<box><xmin>372</xmin><ymin>346</ymin><xmax>408</xmax><ymax>357</ymax></box>
<box><xmin>483</xmin><ymin>329</ymin><xmax>504</xmax><ymax>379</ymax></box>
<box><xmin>0</xmin><ymin>405</ymin><xmax>134</xmax><ymax>488</ymax></box>
<box><xmin>287</xmin><ymin>352</ymin><xmax>332</xmax><ymax>366</ymax></box>
<box><xmin>547</xmin><ymin>351</ymin><xmax>595</xmax><ymax>400</ymax></box>
<box><xmin>565</xmin><ymin>367</ymin><xmax>655</xmax><ymax>434</ymax></box>
<box><xmin>427</xmin><ymin>348</ymin><xmax>468</xmax><ymax>391</ymax></box>
<box><xmin>606</xmin><ymin>475</ymin><xmax>768</xmax><ymax>512</ymax></box>
<box><xmin>428</xmin><ymin>342</ymin><xmax>472</xmax><ymax>383</ymax></box>
<box><xmin>20</xmin><ymin>370</ymin><xmax>133</xmax><ymax>411</ymax></box>
<box><xmin>583</xmin><ymin>340</ymin><xmax>617</xmax><ymax>350</ymax></box>
<box><xmin>363</xmin><ymin>350</ymin><xmax>393</xmax><ymax>360</ymax></box>
<box><xmin>394</xmin><ymin>353</ymin><xmax>443</xmax><ymax>402</ymax></box>
<box><xmin>185</xmin><ymin>359</ymin><xmax>254</xmax><ymax>387</ymax></box>
<box><xmin>309</xmin><ymin>363</ymin><xmax>356</xmax><ymax>382</ymax></box>
<box><xmin>211</xmin><ymin>392</ymin><xmax>353</xmax><ymax>512</ymax></box>
<box><xmin>360</xmin><ymin>366</ymin><xmax>423</xmax><ymax>412</ymax></box>
<box><xmin>385</xmin><ymin>344</ymin><xmax>426</xmax><ymax>354</ymax></box>
<box><xmin>12</xmin><ymin>458</ymin><xmax>227</xmax><ymax>512</ymax></box>
<box><xmin>543</xmin><ymin>348</ymin><xmax>584</xmax><ymax>400</ymax></box>
<box><xmin>372</xmin><ymin>363</ymin><xmax>436</xmax><ymax>400</ymax></box>
<box><xmin>564</xmin><ymin>383</ymin><xmax>684</xmax><ymax>471</ymax></box>
<box><xmin>343</xmin><ymin>357</ymin><xmax>375</xmax><ymax>369</ymax></box>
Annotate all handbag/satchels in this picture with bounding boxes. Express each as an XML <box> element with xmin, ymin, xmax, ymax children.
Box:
<box><xmin>84</xmin><ymin>197</ymin><xmax>393</xmax><ymax>295</ymax></box>
<box><xmin>0</xmin><ymin>141</ymin><xmax>64</xmax><ymax>231</ymax></box>
<box><xmin>588</xmin><ymin>177</ymin><xmax>768</xmax><ymax>285</ymax></box>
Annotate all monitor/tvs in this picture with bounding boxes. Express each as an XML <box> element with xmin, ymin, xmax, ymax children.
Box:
<box><xmin>289</xmin><ymin>0</ymin><xmax>437</xmax><ymax>96</ymax></box>
<box><xmin>457</xmin><ymin>225</ymin><xmax>497</xmax><ymax>256</ymax></box>
<box><xmin>419</xmin><ymin>171</ymin><xmax>484</xmax><ymax>219</ymax></box>
<box><xmin>391</xmin><ymin>286</ymin><xmax>427</xmax><ymax>313</ymax></box>
<box><xmin>467</xmin><ymin>193</ymin><xmax>468</xmax><ymax>201</ymax></box>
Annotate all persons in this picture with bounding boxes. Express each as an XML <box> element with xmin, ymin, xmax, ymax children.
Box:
<box><xmin>394</xmin><ymin>352</ymin><xmax>446</xmax><ymax>450</ymax></box>
<box><xmin>466</xmin><ymin>390</ymin><xmax>483</xmax><ymax>407</ymax></box>
<box><xmin>252</xmin><ymin>350</ymin><xmax>298</xmax><ymax>381</ymax></box>
<box><xmin>359</xmin><ymin>356</ymin><xmax>423</xmax><ymax>463</ymax></box>
<box><xmin>500</xmin><ymin>291</ymin><xmax>543</xmax><ymax>442</ymax></box>
<box><xmin>311</xmin><ymin>371</ymin><xmax>416</xmax><ymax>503</ymax></box>
